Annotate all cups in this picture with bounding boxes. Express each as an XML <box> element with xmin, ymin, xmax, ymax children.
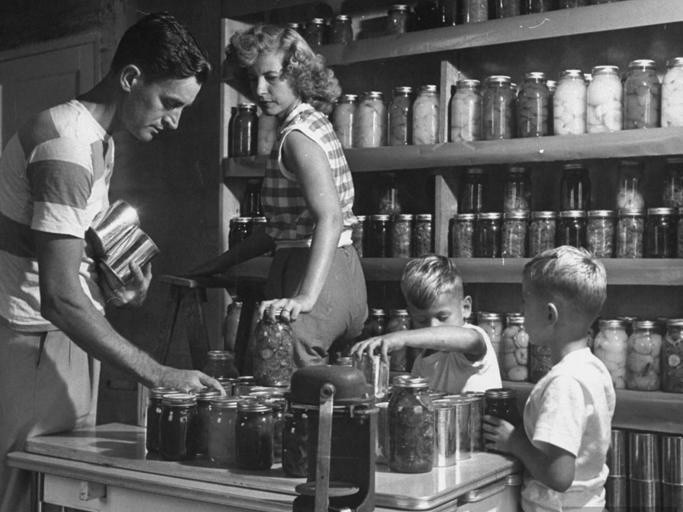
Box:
<box><xmin>604</xmin><ymin>428</ymin><xmax>683</xmax><ymax>512</ymax></box>
<box><xmin>88</xmin><ymin>199</ymin><xmax>160</xmax><ymax>291</ymax></box>
<box><xmin>428</xmin><ymin>388</ymin><xmax>486</xmax><ymax>468</ymax></box>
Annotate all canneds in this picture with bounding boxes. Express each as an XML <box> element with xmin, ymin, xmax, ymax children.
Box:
<box><xmin>450</xmin><ymin>157</ymin><xmax>681</xmax><ymax>259</ymax></box>
<box><xmin>354</xmin><ymin>172</ymin><xmax>434</xmax><ymax>258</ymax></box>
<box><xmin>229</xmin><ymin>178</ymin><xmax>267</xmax><ymax>249</ymax></box>
<box><xmin>327</xmin><ymin>84</ymin><xmax>440</xmax><ymax>149</ymax></box>
<box><xmin>345</xmin><ymin>307</ymin><xmax>428</xmax><ymax>372</ymax></box>
<box><xmin>587</xmin><ymin>315</ymin><xmax>681</xmax><ymax>394</ymax></box>
<box><xmin>385</xmin><ymin>2</ymin><xmax>606</xmax><ymax>38</ymax></box>
<box><xmin>146</xmin><ymin>375</ymin><xmax>514</xmax><ymax>479</ymax></box>
<box><xmin>290</xmin><ymin>14</ymin><xmax>353</xmax><ymax>49</ymax></box>
<box><xmin>468</xmin><ymin>311</ymin><xmax>556</xmax><ymax>384</ymax></box>
<box><xmin>450</xmin><ymin>56</ymin><xmax>681</xmax><ymax>142</ymax></box>
<box><xmin>230</xmin><ymin>102</ymin><xmax>278</xmax><ymax>157</ymax></box>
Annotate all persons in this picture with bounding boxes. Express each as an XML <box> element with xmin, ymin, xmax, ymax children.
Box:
<box><xmin>175</xmin><ymin>18</ymin><xmax>370</xmax><ymax>448</ymax></box>
<box><xmin>346</xmin><ymin>253</ymin><xmax>502</xmax><ymax>450</ymax></box>
<box><xmin>479</xmin><ymin>244</ymin><xmax>616</xmax><ymax>511</ymax></box>
<box><xmin>0</xmin><ymin>0</ymin><xmax>227</xmax><ymax>512</ymax></box>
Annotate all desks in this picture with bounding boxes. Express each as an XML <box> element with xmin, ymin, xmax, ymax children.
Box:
<box><xmin>0</xmin><ymin>419</ymin><xmax>523</xmax><ymax>512</ymax></box>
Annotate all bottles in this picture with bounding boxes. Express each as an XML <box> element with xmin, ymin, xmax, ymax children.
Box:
<box><xmin>373</xmin><ymin>399</ymin><xmax>390</xmax><ymax>464</ymax></box>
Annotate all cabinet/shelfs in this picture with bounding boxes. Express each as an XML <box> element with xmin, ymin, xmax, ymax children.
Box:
<box><xmin>218</xmin><ymin>16</ymin><xmax>683</xmax><ymax>512</ymax></box>
